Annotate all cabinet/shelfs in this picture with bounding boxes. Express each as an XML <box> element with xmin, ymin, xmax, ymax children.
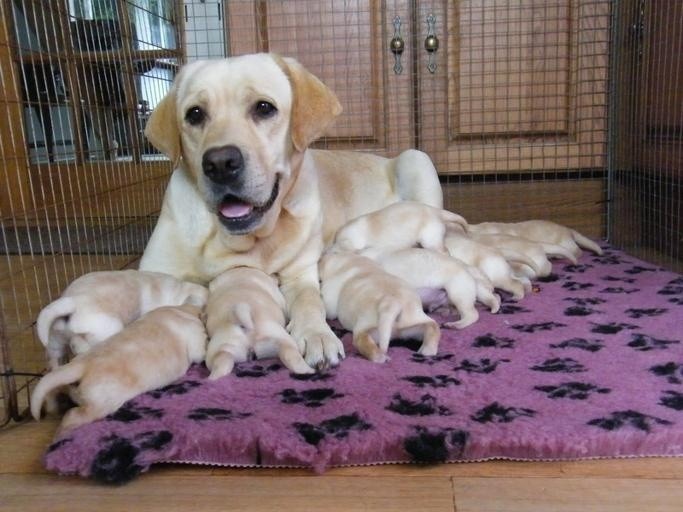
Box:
<box><xmin>220</xmin><ymin>5</ymin><xmax>612</xmax><ymax>189</ymax></box>
<box><xmin>611</xmin><ymin>0</ymin><xmax>682</xmax><ymax>190</ymax></box>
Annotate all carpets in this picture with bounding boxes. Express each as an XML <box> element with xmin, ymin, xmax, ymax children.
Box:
<box><xmin>39</xmin><ymin>232</ymin><xmax>683</xmax><ymax>485</ymax></box>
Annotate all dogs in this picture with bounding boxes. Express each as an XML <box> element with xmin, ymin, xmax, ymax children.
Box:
<box><xmin>332</xmin><ymin>198</ymin><xmax>469</xmax><ymax>249</ymax></box>
<box><xmin>137</xmin><ymin>52</ymin><xmax>451</xmax><ymax>373</ymax></box>
<box><xmin>28</xmin><ymin>304</ymin><xmax>213</xmax><ymax>430</ymax></box>
<box><xmin>204</xmin><ymin>267</ymin><xmax>318</xmax><ymax>382</ymax></box>
<box><xmin>444</xmin><ymin>229</ymin><xmax>534</xmax><ymax>303</ymax></box>
<box><xmin>35</xmin><ymin>268</ymin><xmax>209</xmax><ymax>418</ymax></box>
<box><xmin>469</xmin><ymin>232</ymin><xmax>581</xmax><ymax>279</ymax></box>
<box><xmin>359</xmin><ymin>247</ymin><xmax>503</xmax><ymax>329</ymax></box>
<box><xmin>316</xmin><ymin>248</ymin><xmax>444</xmax><ymax>367</ymax></box>
<box><xmin>470</xmin><ymin>219</ymin><xmax>604</xmax><ymax>269</ymax></box>
